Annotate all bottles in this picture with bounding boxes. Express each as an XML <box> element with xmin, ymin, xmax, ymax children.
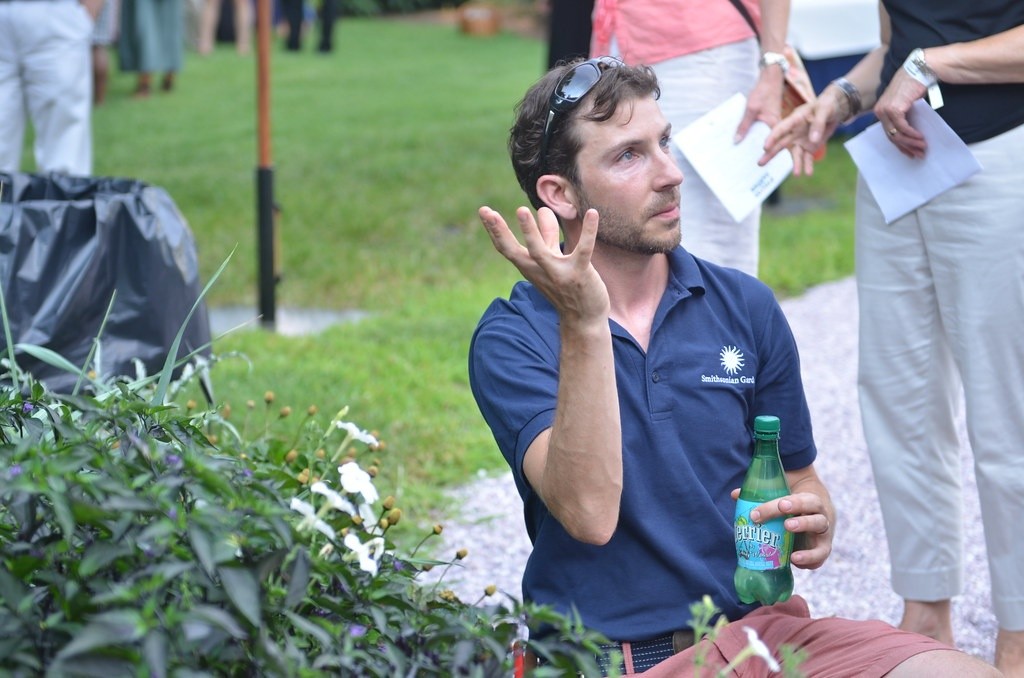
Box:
<box><xmin>734</xmin><ymin>413</ymin><xmax>795</xmax><ymax>606</ymax></box>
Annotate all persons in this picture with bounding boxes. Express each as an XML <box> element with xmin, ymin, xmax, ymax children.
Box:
<box><xmin>543</xmin><ymin>0</ymin><xmax>791</xmax><ymax>278</ymax></box>
<box><xmin>0</xmin><ymin>0</ymin><xmax>105</xmax><ymax>179</ymax></box>
<box><xmin>757</xmin><ymin>0</ymin><xmax>1023</xmax><ymax>678</ymax></box>
<box><xmin>469</xmin><ymin>57</ymin><xmax>1005</xmax><ymax>678</ymax></box>
<box><xmin>80</xmin><ymin>0</ymin><xmax>337</xmax><ymax>103</ymax></box>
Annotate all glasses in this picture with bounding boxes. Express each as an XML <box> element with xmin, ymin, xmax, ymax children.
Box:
<box><xmin>544</xmin><ymin>55</ymin><xmax>628</xmax><ymax>171</ymax></box>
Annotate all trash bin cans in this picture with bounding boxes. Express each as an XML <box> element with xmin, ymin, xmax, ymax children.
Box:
<box><xmin>0</xmin><ymin>171</ymin><xmax>213</xmax><ymax>399</ymax></box>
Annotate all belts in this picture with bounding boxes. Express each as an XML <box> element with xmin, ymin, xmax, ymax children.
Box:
<box><xmin>542</xmin><ymin>628</ymin><xmax>702</xmax><ymax>678</ymax></box>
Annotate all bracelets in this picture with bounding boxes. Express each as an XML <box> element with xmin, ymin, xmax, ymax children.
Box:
<box><xmin>758</xmin><ymin>52</ymin><xmax>788</xmax><ymax>75</ymax></box>
<box><xmin>904</xmin><ymin>49</ymin><xmax>937</xmax><ymax>88</ymax></box>
<box><xmin>830</xmin><ymin>78</ymin><xmax>861</xmax><ymax>123</ymax></box>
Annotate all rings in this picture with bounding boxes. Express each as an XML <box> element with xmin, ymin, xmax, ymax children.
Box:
<box><xmin>818</xmin><ymin>515</ymin><xmax>831</xmax><ymax>535</ymax></box>
<box><xmin>887</xmin><ymin>129</ymin><xmax>896</xmax><ymax>136</ymax></box>
